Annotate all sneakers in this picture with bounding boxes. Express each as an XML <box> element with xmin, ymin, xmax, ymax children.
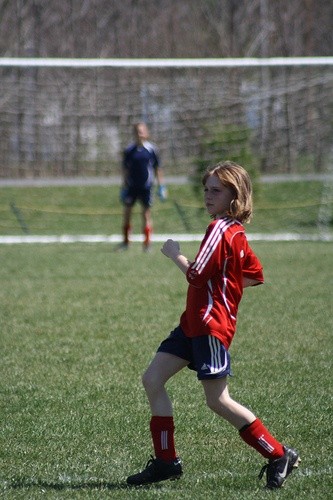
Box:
<box><xmin>127</xmin><ymin>454</ymin><xmax>183</xmax><ymax>487</ymax></box>
<box><xmin>259</xmin><ymin>446</ymin><xmax>302</xmax><ymax>489</ymax></box>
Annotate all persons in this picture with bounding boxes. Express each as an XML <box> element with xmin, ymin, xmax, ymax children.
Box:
<box><xmin>115</xmin><ymin>122</ymin><xmax>169</xmax><ymax>253</ymax></box>
<box><xmin>126</xmin><ymin>160</ymin><xmax>302</xmax><ymax>491</ymax></box>
